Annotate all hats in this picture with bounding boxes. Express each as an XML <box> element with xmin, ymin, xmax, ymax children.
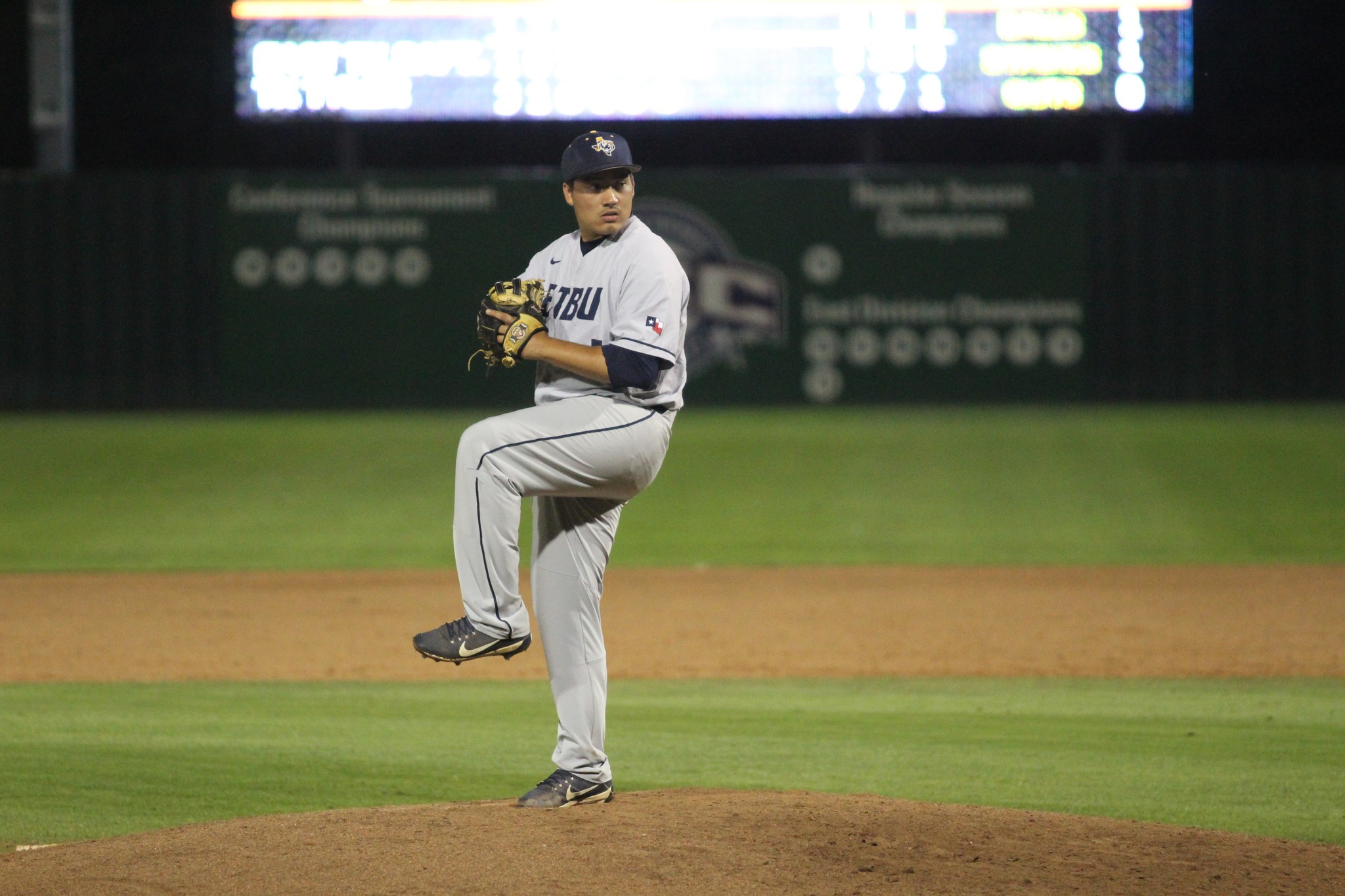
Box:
<box><xmin>559</xmin><ymin>130</ymin><xmax>642</xmax><ymax>183</ymax></box>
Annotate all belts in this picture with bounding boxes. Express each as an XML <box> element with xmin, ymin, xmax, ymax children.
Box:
<box><xmin>650</xmin><ymin>403</ymin><xmax>667</xmax><ymax>415</ymax></box>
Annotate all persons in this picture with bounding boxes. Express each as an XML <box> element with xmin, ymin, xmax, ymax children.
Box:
<box><xmin>414</xmin><ymin>129</ymin><xmax>691</xmax><ymax>810</ymax></box>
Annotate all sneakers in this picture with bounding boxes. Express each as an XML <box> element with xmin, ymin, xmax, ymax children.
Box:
<box><xmin>412</xmin><ymin>616</ymin><xmax>533</xmax><ymax>666</ymax></box>
<box><xmin>517</xmin><ymin>773</ymin><xmax>614</xmax><ymax>809</ymax></box>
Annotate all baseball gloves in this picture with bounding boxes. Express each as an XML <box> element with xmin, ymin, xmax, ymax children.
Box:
<box><xmin>477</xmin><ymin>277</ymin><xmax>544</xmax><ymax>371</ymax></box>
